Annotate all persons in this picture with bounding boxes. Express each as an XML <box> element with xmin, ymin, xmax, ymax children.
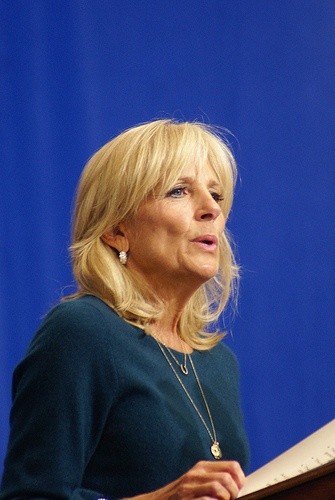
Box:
<box><xmin>0</xmin><ymin>119</ymin><xmax>254</xmax><ymax>500</ymax></box>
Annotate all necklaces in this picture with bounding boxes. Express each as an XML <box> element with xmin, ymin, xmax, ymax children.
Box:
<box><xmin>144</xmin><ymin>322</ymin><xmax>223</xmax><ymax>461</ymax></box>
<box><xmin>140</xmin><ymin>320</ymin><xmax>190</xmax><ymax>375</ymax></box>
